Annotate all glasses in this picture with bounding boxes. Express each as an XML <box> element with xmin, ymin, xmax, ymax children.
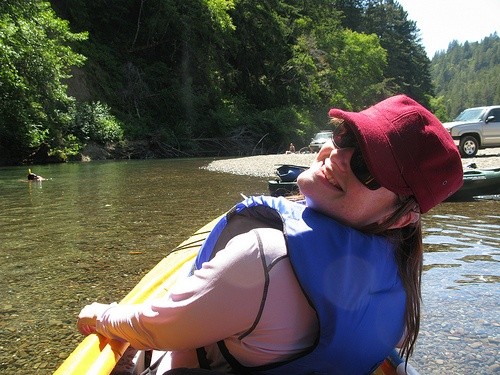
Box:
<box><xmin>331</xmin><ymin>120</ymin><xmax>381</xmax><ymax>190</ymax></box>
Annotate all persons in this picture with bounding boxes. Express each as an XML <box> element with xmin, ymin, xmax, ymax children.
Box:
<box><xmin>76</xmin><ymin>92</ymin><xmax>464</xmax><ymax>375</ymax></box>
<box><xmin>22</xmin><ymin>173</ymin><xmax>46</xmax><ymax>182</ymax></box>
<box><xmin>284</xmin><ymin>142</ymin><xmax>296</xmax><ymax>154</ymax></box>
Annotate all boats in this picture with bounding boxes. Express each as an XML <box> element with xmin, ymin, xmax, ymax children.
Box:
<box><xmin>269</xmin><ymin>162</ymin><xmax>500</xmax><ymax>200</ymax></box>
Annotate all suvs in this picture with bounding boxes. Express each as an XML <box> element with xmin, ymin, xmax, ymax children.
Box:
<box><xmin>310</xmin><ymin>130</ymin><xmax>334</xmax><ymax>153</ymax></box>
<box><xmin>442</xmin><ymin>105</ymin><xmax>500</xmax><ymax>158</ymax></box>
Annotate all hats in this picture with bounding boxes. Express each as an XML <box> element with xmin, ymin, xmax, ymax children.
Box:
<box><xmin>329</xmin><ymin>94</ymin><xmax>464</xmax><ymax>214</ymax></box>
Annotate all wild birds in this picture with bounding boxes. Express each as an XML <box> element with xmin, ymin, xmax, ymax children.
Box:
<box><xmin>27</xmin><ymin>168</ymin><xmax>43</xmax><ymax>180</ymax></box>
<box><xmin>27</xmin><ymin>180</ymin><xmax>43</xmax><ymax>193</ymax></box>
